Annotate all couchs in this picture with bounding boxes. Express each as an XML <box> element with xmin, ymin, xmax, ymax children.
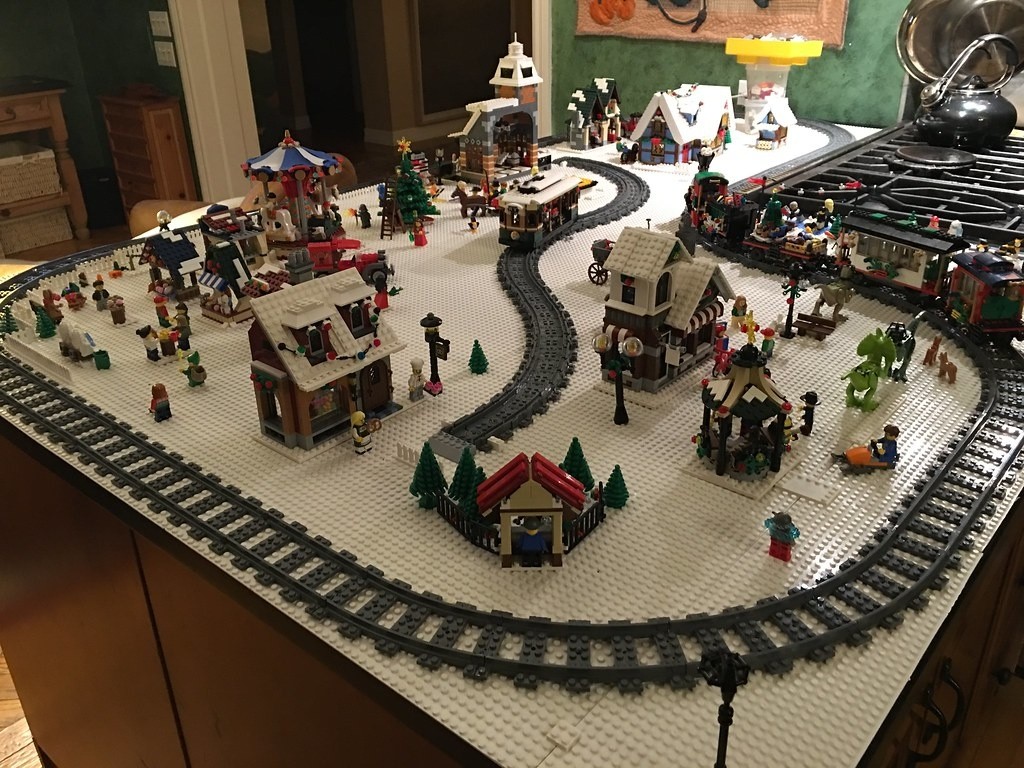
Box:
<box><xmin>129</xmin><ymin>153</ymin><xmax>358</xmax><ymax>241</ymax></box>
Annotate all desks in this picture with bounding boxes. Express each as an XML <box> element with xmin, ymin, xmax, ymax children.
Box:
<box><xmin>0</xmin><ymin>73</ymin><xmax>91</xmax><ymax>241</ymax></box>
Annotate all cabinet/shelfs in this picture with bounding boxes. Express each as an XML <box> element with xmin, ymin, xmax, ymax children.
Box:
<box><xmin>865</xmin><ymin>493</ymin><xmax>1024</xmax><ymax>767</ymax></box>
<box><xmin>96</xmin><ymin>85</ymin><xmax>198</xmax><ymax>234</ymax></box>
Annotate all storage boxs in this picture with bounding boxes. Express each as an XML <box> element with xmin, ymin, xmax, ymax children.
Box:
<box><xmin>0</xmin><ymin>206</ymin><xmax>74</xmax><ymax>257</ymax></box>
<box><xmin>0</xmin><ymin>139</ymin><xmax>62</xmax><ymax>205</ymax></box>
